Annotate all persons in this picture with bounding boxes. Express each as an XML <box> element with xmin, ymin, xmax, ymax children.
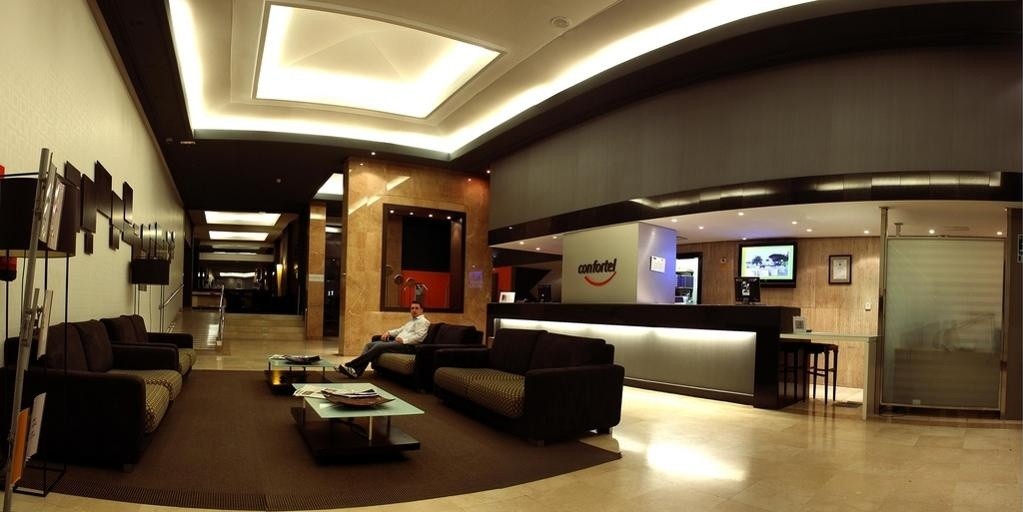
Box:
<box><xmin>333</xmin><ymin>301</ymin><xmax>431</xmax><ymax>380</ymax></box>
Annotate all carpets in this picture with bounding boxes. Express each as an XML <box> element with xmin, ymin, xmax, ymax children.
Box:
<box><xmin>17</xmin><ymin>366</ymin><xmax>622</xmax><ymax>511</ymax></box>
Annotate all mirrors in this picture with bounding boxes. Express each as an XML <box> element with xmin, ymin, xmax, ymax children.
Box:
<box><xmin>386</xmin><ymin>206</ymin><xmax>462</xmax><ymax>308</ymax></box>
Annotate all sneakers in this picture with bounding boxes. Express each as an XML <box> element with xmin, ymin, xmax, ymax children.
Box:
<box><xmin>339</xmin><ymin>364</ymin><xmax>358</xmax><ymax>379</ymax></box>
<box><xmin>333</xmin><ymin>365</ymin><xmax>346</xmax><ymax>373</ymax></box>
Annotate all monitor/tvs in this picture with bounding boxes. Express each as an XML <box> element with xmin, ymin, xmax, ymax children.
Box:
<box><xmin>737</xmin><ymin>241</ymin><xmax>797</xmax><ymax>288</ymax></box>
<box><xmin>734</xmin><ymin>276</ymin><xmax>761</xmax><ymax>304</ymax></box>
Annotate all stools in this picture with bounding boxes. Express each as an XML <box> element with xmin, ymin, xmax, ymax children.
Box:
<box><xmin>804</xmin><ymin>344</ymin><xmax>838</xmax><ymax>405</ymax></box>
<box><xmin>778</xmin><ymin>338</ymin><xmax>811</xmax><ymax>404</ymax></box>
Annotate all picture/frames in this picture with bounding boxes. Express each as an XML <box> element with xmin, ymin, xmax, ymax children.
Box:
<box><xmin>828</xmin><ymin>254</ymin><xmax>853</xmax><ymax>286</ymax></box>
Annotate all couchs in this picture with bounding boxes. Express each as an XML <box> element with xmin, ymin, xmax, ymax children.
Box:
<box><xmin>371</xmin><ymin>321</ymin><xmax>488</xmax><ymax>392</ymax></box>
<box><xmin>99</xmin><ymin>314</ymin><xmax>197</xmax><ymax>383</ymax></box>
<box><xmin>433</xmin><ymin>327</ymin><xmax>625</xmax><ymax>441</ymax></box>
<box><xmin>6</xmin><ymin>319</ymin><xmax>183</xmax><ymax>474</ymax></box>
